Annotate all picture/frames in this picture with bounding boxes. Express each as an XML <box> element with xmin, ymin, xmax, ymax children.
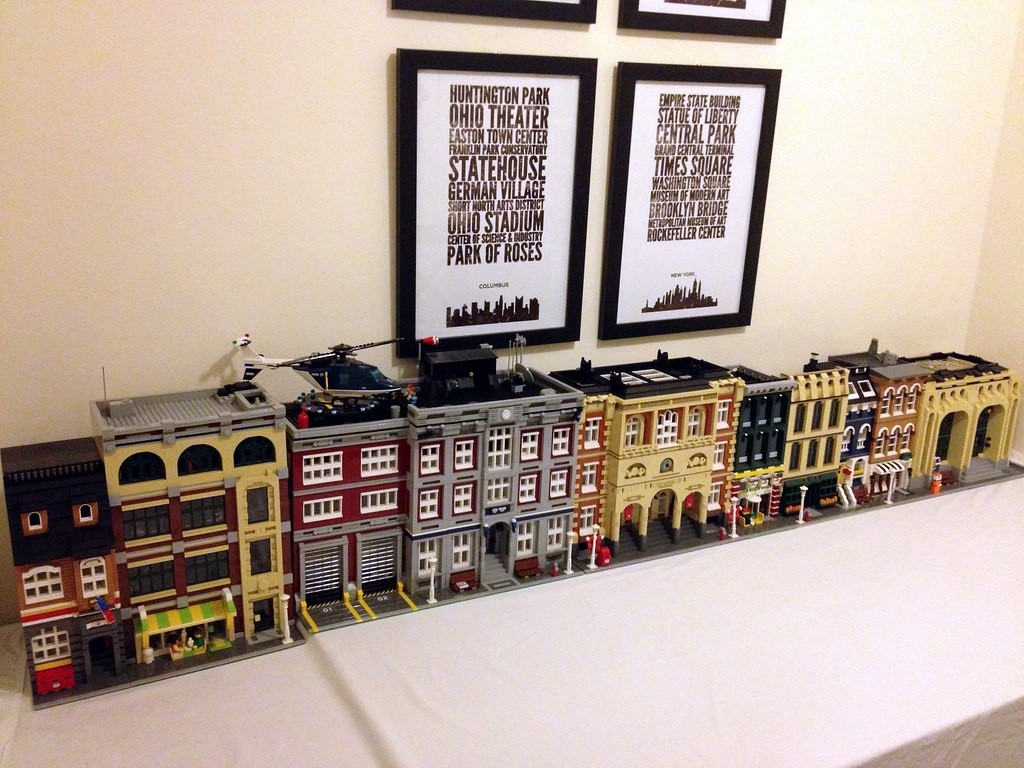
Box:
<box><xmin>617</xmin><ymin>0</ymin><xmax>787</xmax><ymax>39</ymax></box>
<box><xmin>597</xmin><ymin>64</ymin><xmax>782</xmax><ymax>341</ymax></box>
<box><xmin>394</xmin><ymin>48</ymin><xmax>598</xmax><ymax>359</ymax></box>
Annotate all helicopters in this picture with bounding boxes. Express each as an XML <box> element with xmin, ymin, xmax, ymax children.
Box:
<box><xmin>232</xmin><ymin>332</ymin><xmax>408</xmax><ymax>413</ymax></box>
